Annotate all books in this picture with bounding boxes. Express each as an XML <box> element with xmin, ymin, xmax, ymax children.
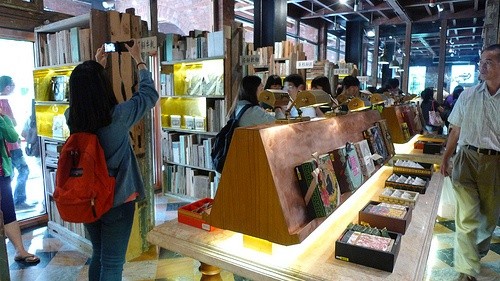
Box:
<box><xmin>306</xmin><ymin>60</ymin><xmax>325</xmax><ymax>78</ymax></box>
<box><xmin>50</xmin><ymin>201</ymin><xmax>86</xmax><ymax>240</ymax></box>
<box><xmin>45</xmin><ymin>140</ymin><xmax>60</xmax><ymax>169</ymax></box>
<box><xmin>256</xmin><ymin>71</ymin><xmax>269</xmax><ymax>86</ymax></box>
<box><xmin>163</xmin><ymin>131</ymin><xmax>215</xmax><ymax>169</ymax></box>
<box><xmin>46</xmin><ymin>170</ymin><xmax>56</xmax><ymax>194</ymax></box>
<box><xmin>338</xmin><ymin>62</ymin><xmax>353</xmax><ymax>73</ymax></box>
<box><xmin>275</xmin><ymin>60</ymin><xmax>290</xmax><ymax>77</ymax></box>
<box><xmin>208</xmin><ymin>99</ymin><xmax>224</xmax><ymax>132</ymax></box>
<box><xmin>50</xmin><ymin>76</ymin><xmax>69</xmax><ymax>101</ymax></box>
<box><xmin>254</xmin><ymin>46</ymin><xmax>273</xmax><ymax>64</ymax></box>
<box><xmin>164</xmin><ymin>163</ymin><xmax>216</xmax><ymax>199</ymax></box>
<box><xmin>160</xmin><ymin>29</ymin><xmax>224</xmax><ymax>62</ymax></box>
<box><xmin>274</xmin><ymin>41</ymin><xmax>297</xmax><ymax>59</ymax></box>
<box><xmin>38</xmin><ymin>27</ymin><xmax>90</xmax><ymax>67</ymax></box>
<box><xmin>160</xmin><ymin>72</ymin><xmax>173</xmax><ymax>95</ymax></box>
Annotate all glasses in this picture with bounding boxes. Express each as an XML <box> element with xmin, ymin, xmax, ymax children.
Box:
<box><xmin>283</xmin><ymin>86</ymin><xmax>297</xmax><ymax>90</ymax></box>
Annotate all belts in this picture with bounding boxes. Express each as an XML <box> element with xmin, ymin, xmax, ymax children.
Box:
<box><xmin>465</xmin><ymin>144</ymin><xmax>500</xmax><ymax>155</ymax></box>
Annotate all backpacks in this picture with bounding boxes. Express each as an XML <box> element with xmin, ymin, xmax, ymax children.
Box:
<box><xmin>52</xmin><ymin>133</ymin><xmax>115</xmax><ymax>223</ymax></box>
<box><xmin>21</xmin><ymin>117</ymin><xmax>40</xmax><ymax>158</ymax></box>
<box><xmin>210</xmin><ymin>103</ymin><xmax>260</xmax><ymax>174</ymax></box>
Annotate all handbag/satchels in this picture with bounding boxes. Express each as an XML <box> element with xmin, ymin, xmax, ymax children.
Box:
<box><xmin>429</xmin><ymin>100</ymin><xmax>444</xmax><ymax>126</ymax></box>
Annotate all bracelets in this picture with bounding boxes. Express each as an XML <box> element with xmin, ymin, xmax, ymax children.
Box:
<box><xmin>273</xmin><ymin>106</ymin><xmax>283</xmax><ymax>112</ymax></box>
<box><xmin>136</xmin><ymin>62</ymin><xmax>146</xmax><ymax>68</ymax></box>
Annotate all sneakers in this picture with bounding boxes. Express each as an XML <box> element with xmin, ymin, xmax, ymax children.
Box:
<box><xmin>14</xmin><ymin>202</ymin><xmax>38</xmax><ymax>213</ymax></box>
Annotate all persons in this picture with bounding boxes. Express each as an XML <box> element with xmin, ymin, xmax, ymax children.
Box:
<box><xmin>0</xmin><ymin>108</ymin><xmax>41</xmax><ymax>266</ymax></box>
<box><xmin>21</xmin><ymin>114</ymin><xmax>47</xmax><ymax>214</ymax></box>
<box><xmin>225</xmin><ymin>74</ymin><xmax>405</xmax><ymax>127</ymax></box>
<box><xmin>0</xmin><ymin>76</ymin><xmax>39</xmax><ymax>211</ymax></box>
<box><xmin>441</xmin><ymin>43</ymin><xmax>500</xmax><ymax>281</ymax></box>
<box><xmin>64</xmin><ymin>38</ymin><xmax>159</xmax><ymax>281</ymax></box>
<box><xmin>420</xmin><ymin>82</ymin><xmax>464</xmax><ymax>134</ymax></box>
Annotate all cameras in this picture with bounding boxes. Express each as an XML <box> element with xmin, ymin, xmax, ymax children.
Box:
<box><xmin>103</xmin><ymin>40</ymin><xmax>135</xmax><ymax>53</ymax></box>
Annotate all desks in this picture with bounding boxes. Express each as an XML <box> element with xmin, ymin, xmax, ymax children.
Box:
<box><xmin>145</xmin><ymin>100</ymin><xmax>453</xmax><ymax>281</ymax></box>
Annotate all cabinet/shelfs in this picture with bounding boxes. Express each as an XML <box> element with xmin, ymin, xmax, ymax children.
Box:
<box><xmin>158</xmin><ymin>31</ymin><xmax>369</xmax><ymax>204</ymax></box>
<box><xmin>33</xmin><ymin>8</ymin><xmax>155</xmax><ymax>262</ymax></box>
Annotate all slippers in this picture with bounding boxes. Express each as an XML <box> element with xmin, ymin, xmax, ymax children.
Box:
<box><xmin>14</xmin><ymin>254</ymin><xmax>40</xmax><ymax>265</ymax></box>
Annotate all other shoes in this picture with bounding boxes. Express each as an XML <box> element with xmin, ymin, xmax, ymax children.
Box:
<box><xmin>454</xmin><ymin>273</ymin><xmax>477</xmax><ymax>281</ymax></box>
